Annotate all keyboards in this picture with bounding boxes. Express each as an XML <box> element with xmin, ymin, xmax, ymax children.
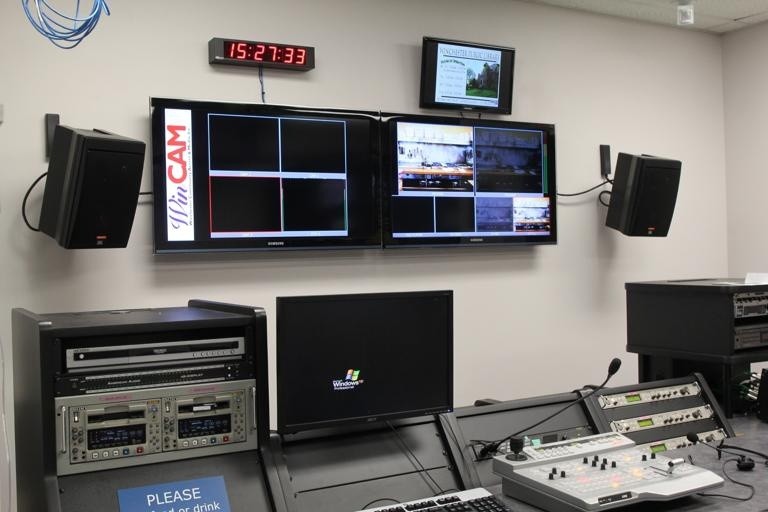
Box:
<box><xmin>350</xmin><ymin>488</ymin><xmax>513</xmax><ymax>512</ymax></box>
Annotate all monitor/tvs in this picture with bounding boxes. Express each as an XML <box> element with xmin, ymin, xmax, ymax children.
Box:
<box><xmin>277</xmin><ymin>288</ymin><xmax>453</xmax><ymax>436</ymax></box>
<box><xmin>382</xmin><ymin>115</ymin><xmax>558</xmax><ymax>247</ymax></box>
<box><xmin>148</xmin><ymin>97</ymin><xmax>382</xmax><ymax>254</ymax></box>
<box><xmin>418</xmin><ymin>36</ymin><xmax>516</xmax><ymax>115</ymax></box>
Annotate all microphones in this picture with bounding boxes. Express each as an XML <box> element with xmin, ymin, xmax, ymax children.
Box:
<box><xmin>687</xmin><ymin>431</ymin><xmax>756</xmax><ymax>471</ymax></box>
<box><xmin>481</xmin><ymin>357</ymin><xmax>622</xmax><ymax>458</ymax></box>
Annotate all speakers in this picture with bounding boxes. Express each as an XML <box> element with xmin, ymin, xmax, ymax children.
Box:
<box><xmin>38</xmin><ymin>126</ymin><xmax>147</xmax><ymax>249</ymax></box>
<box><xmin>606</xmin><ymin>152</ymin><xmax>681</xmax><ymax>238</ymax></box>
